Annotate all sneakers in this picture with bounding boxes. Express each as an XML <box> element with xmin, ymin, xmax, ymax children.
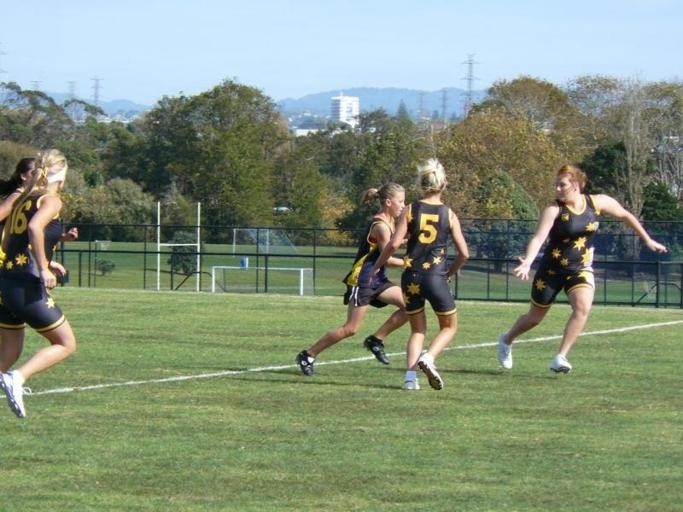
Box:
<box><xmin>294</xmin><ymin>349</ymin><xmax>317</xmax><ymax>376</ymax></box>
<box><xmin>498</xmin><ymin>332</ymin><xmax>513</xmax><ymax>369</ymax></box>
<box><xmin>363</xmin><ymin>334</ymin><xmax>390</xmax><ymax>364</ymax></box>
<box><xmin>549</xmin><ymin>353</ymin><xmax>572</xmax><ymax>373</ymax></box>
<box><xmin>0</xmin><ymin>369</ymin><xmax>31</xmax><ymax>418</ymax></box>
<box><xmin>402</xmin><ymin>355</ymin><xmax>444</xmax><ymax>390</ymax></box>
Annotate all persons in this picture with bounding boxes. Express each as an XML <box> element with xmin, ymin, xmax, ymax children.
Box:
<box><xmin>498</xmin><ymin>164</ymin><xmax>667</xmax><ymax>373</ymax></box>
<box><xmin>367</xmin><ymin>158</ymin><xmax>469</xmax><ymax>390</ymax></box>
<box><xmin>0</xmin><ymin>158</ymin><xmax>78</xmax><ymax>242</ymax></box>
<box><xmin>0</xmin><ymin>147</ymin><xmax>76</xmax><ymax>417</ymax></box>
<box><xmin>295</xmin><ymin>183</ymin><xmax>409</xmax><ymax>376</ymax></box>
